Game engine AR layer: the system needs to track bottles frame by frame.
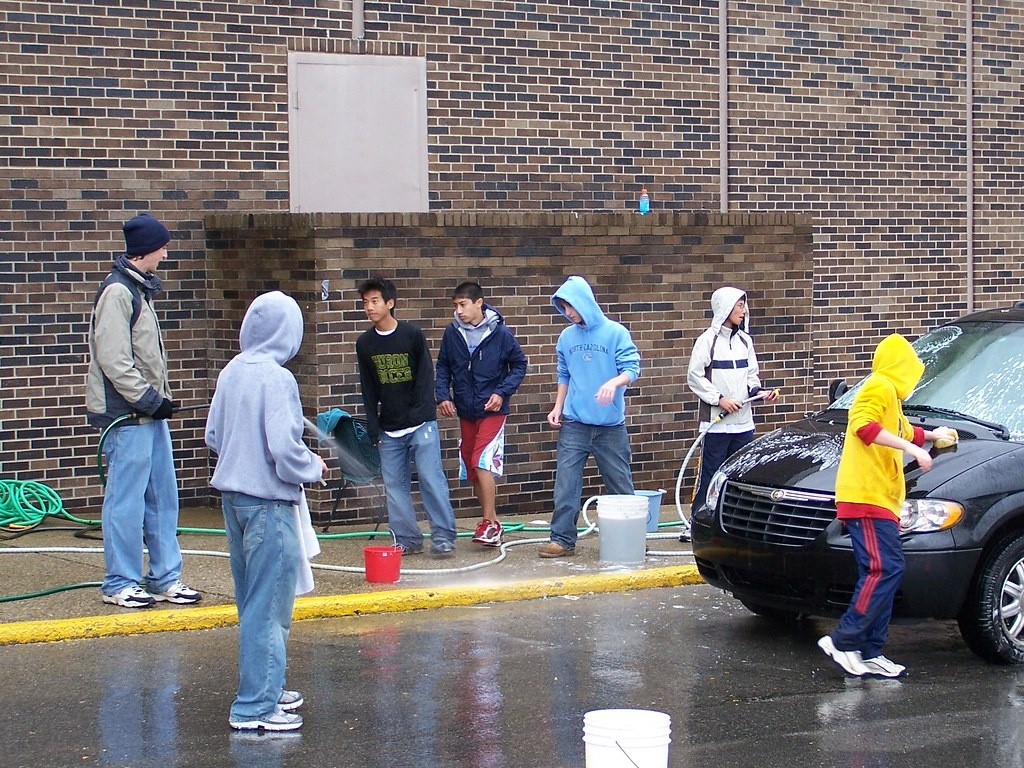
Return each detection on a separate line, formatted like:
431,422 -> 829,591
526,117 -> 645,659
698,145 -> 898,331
640,189 -> 649,213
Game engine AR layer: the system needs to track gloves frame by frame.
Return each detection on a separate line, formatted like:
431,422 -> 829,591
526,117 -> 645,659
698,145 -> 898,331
152,398 -> 180,420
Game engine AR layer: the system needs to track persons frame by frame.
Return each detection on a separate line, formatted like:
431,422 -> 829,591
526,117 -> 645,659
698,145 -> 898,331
86,213 -> 202,608
436,281 -> 527,547
679,287 -> 779,542
817,334 -> 956,677
204,290 -> 328,730
356,279 -> 456,557
538,276 -> 650,557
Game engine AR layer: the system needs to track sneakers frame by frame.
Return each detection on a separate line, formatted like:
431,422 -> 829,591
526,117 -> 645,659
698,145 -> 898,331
817,634 -> 871,676
390,539 -> 424,556
539,541 -> 576,559
275,688 -> 304,711
431,537 -> 455,554
861,653 -> 909,679
144,578 -> 204,605
101,583 -> 156,609
229,701 -> 304,730
471,518 -> 505,547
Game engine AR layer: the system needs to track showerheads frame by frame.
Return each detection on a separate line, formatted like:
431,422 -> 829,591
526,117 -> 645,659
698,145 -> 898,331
716,389 -> 781,422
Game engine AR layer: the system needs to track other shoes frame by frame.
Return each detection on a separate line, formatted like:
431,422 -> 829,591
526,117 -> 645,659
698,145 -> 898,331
679,526 -> 692,542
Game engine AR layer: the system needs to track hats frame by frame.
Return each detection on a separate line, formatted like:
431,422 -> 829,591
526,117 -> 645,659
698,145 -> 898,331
123,213 -> 171,256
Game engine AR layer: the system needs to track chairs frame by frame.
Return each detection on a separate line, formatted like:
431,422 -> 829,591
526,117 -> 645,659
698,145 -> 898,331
315,408 -> 387,541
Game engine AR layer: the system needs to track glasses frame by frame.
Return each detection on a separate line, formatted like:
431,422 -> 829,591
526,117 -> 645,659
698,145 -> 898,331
736,303 -> 746,309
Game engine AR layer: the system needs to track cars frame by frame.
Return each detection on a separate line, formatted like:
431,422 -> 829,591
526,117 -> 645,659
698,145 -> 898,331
688,300 -> 1024,667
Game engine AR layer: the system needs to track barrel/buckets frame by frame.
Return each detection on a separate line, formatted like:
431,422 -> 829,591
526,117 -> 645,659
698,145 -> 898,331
582,708 -> 672,768
596,494 -> 649,564
363,527 -> 403,584
633,487 -> 668,533
